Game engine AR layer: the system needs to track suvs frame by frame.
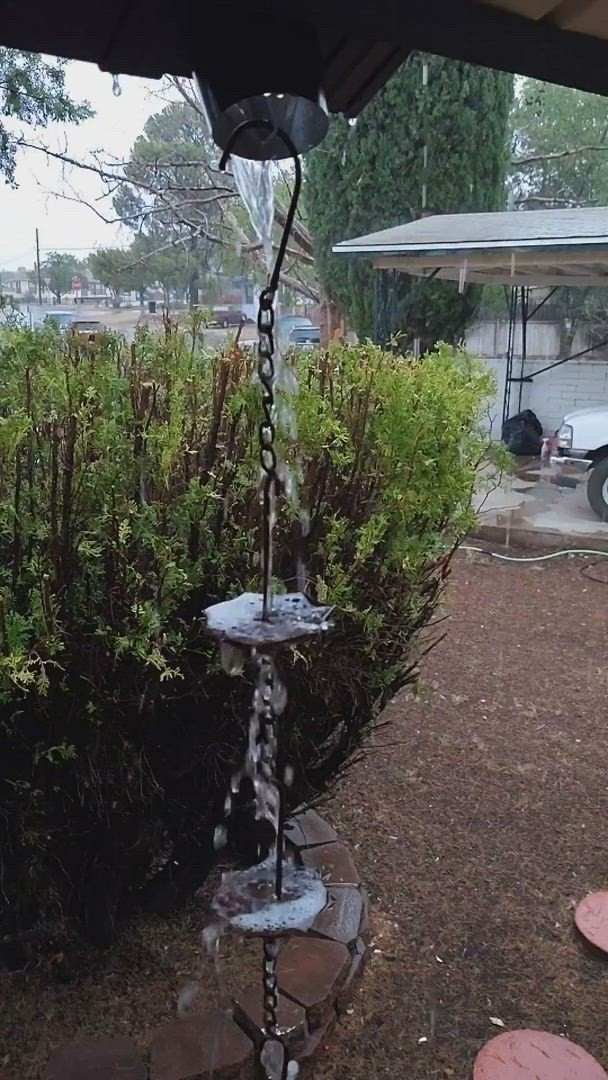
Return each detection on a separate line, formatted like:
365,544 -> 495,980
541,404 -> 608,520
288,324 -> 321,347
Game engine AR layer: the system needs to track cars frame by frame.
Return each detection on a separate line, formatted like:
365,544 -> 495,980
275,315 -> 312,340
207,304 -> 247,328
68,320 -> 104,344
34,310 -> 77,337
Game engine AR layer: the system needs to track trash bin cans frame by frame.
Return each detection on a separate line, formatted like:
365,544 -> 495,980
149,301 -> 156,313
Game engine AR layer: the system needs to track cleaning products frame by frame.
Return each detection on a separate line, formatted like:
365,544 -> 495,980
540,437 -> 549,460
